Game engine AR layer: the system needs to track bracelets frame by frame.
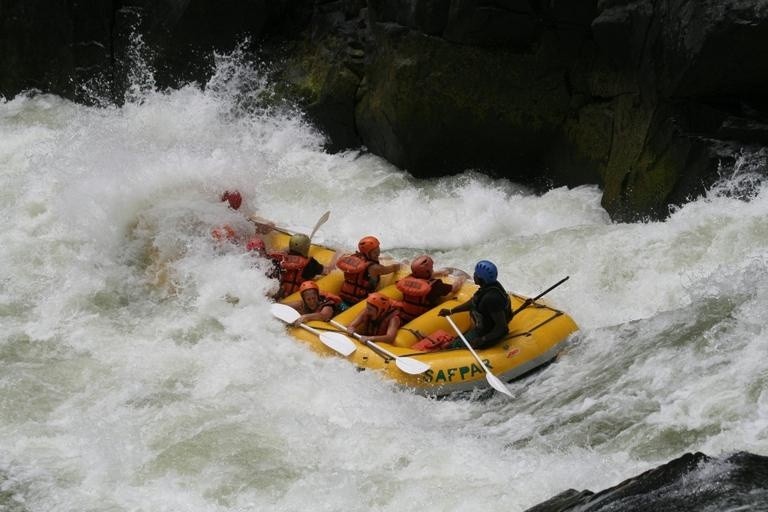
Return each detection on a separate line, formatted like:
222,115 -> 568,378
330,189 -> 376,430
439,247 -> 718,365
450,308 -> 452,314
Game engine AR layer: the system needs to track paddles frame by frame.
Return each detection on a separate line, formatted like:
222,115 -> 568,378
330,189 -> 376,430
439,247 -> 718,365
446,317 -> 517,398
300,324 -> 357,357
271,302 -> 301,323
308,211 -> 331,242
330,320 -> 431,374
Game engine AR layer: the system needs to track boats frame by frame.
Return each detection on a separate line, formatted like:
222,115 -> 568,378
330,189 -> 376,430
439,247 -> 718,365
246,216 -> 580,400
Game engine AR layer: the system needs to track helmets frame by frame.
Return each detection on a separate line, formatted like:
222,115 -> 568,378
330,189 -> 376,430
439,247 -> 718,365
411,255 -> 433,278
211,223 -> 235,242
222,187 -> 241,210
475,260 -> 498,283
287,233 -> 310,256
246,237 -> 266,250
358,236 -> 380,259
366,292 -> 389,319
299,280 -> 319,306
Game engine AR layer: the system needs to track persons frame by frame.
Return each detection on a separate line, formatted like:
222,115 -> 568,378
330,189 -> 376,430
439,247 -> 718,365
282,280 -> 344,328
210,190 -> 340,301
394,254 -> 465,321
335,235 -> 409,307
438,259 -> 516,350
346,292 -> 403,344
